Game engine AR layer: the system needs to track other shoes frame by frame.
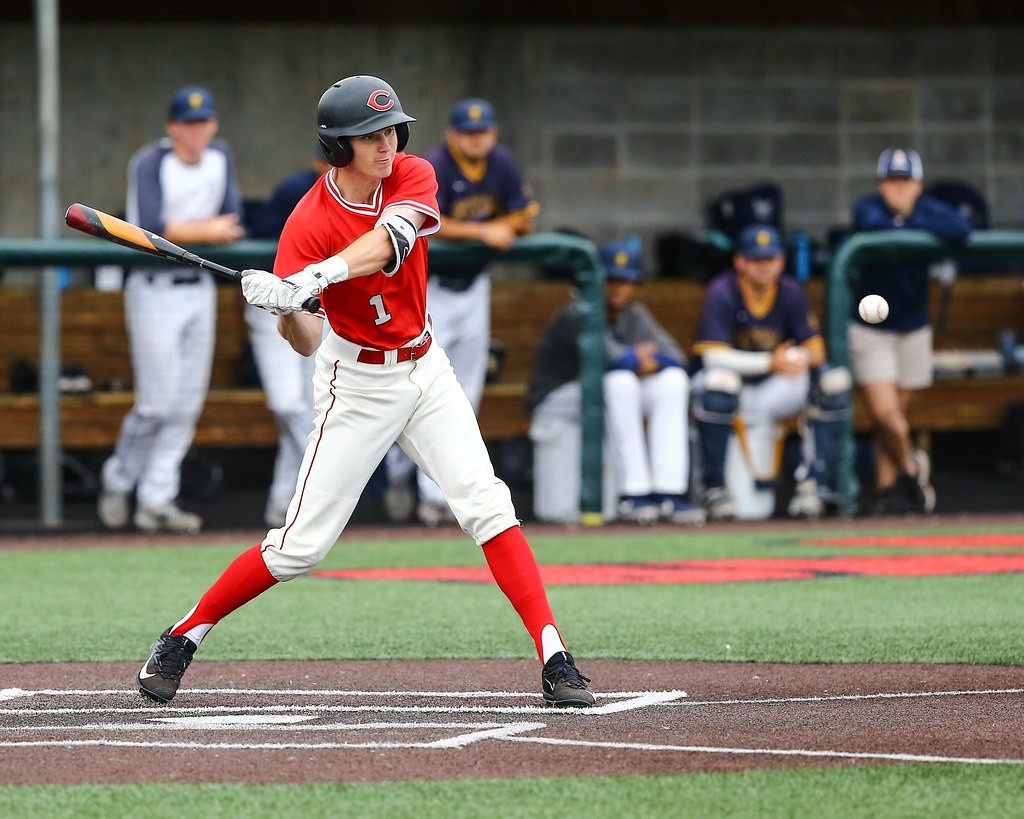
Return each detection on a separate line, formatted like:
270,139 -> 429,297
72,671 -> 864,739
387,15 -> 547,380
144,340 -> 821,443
134,505 -> 202,533
870,490 -> 909,516
905,451 -> 935,512
660,496 -> 708,523
705,488 -> 736,519
619,497 -> 660,521
98,458 -> 132,526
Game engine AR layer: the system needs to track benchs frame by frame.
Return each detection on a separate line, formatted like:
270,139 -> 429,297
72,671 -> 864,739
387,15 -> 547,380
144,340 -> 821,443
0,275 -> 1024,454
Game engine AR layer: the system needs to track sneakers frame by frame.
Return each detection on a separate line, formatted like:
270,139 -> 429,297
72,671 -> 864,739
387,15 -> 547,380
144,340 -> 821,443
542,651 -> 597,705
136,624 -> 197,704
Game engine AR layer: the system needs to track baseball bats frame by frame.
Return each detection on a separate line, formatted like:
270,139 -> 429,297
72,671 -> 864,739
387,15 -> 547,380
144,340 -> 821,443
63,200 -> 322,314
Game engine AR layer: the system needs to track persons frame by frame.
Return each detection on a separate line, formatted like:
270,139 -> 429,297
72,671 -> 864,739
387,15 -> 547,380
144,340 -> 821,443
244,145 -> 332,528
693,223 -> 825,520
97,85 -> 241,534
523,236 -> 707,525
387,98 -> 540,524
825,147 -> 974,515
137,75 -> 596,711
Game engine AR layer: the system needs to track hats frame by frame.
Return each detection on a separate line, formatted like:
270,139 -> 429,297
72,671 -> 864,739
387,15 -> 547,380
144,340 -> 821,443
738,226 -> 784,258
451,98 -> 495,131
170,87 -> 215,121
602,243 -> 647,282
877,149 -> 925,182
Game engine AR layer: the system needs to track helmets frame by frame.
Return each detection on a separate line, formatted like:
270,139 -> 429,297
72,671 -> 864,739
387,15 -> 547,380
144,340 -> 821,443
315,75 -> 417,167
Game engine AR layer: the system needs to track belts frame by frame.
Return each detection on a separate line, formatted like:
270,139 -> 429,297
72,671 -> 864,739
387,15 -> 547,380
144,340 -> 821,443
174,277 -> 199,284
440,279 -> 472,291
357,315 -> 432,365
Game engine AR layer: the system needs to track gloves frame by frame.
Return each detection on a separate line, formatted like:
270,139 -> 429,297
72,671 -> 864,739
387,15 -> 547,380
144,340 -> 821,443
240,270 -> 292,315
259,254 -> 349,312
